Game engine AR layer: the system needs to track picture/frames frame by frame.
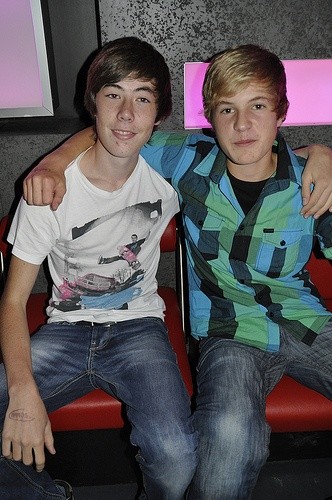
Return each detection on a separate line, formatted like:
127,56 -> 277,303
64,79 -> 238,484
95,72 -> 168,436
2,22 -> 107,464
0,0 -> 54,118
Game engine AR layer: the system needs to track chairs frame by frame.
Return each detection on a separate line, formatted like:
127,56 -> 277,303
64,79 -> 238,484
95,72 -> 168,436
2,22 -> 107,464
0,212 -> 194,435
267,237 -> 332,432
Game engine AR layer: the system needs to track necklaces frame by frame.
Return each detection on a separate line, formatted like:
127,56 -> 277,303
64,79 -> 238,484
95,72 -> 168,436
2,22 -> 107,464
22,44 -> 332,499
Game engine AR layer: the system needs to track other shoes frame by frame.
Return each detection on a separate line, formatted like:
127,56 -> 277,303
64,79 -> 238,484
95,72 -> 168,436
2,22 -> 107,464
54,478 -> 74,499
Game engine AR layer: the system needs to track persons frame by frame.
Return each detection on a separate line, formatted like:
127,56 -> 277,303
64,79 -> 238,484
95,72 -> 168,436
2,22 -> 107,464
0,37 -> 332,500
